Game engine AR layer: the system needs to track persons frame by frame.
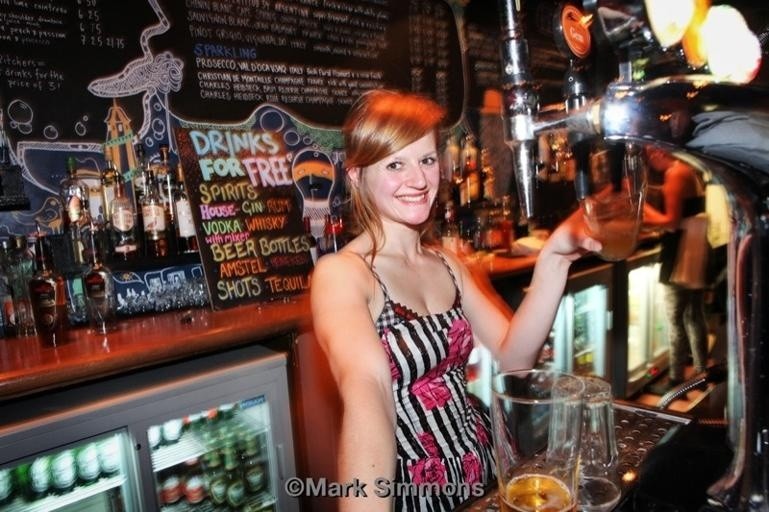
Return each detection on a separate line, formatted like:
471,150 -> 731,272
308,87 -> 613,512
624,144 -> 710,402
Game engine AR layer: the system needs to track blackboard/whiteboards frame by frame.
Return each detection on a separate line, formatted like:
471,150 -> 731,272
2,1 -> 644,214
170,128 -> 324,307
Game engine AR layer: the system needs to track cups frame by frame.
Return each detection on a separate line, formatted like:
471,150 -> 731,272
574,140 -> 648,263
487,368 -> 622,512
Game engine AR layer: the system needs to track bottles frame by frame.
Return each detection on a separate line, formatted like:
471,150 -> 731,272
0,141 -> 198,348
437,135 -> 528,251
533,131 -> 574,185
0,402 -> 276,512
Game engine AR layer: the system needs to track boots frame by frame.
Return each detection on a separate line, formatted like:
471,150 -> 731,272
647,378 -> 686,400
